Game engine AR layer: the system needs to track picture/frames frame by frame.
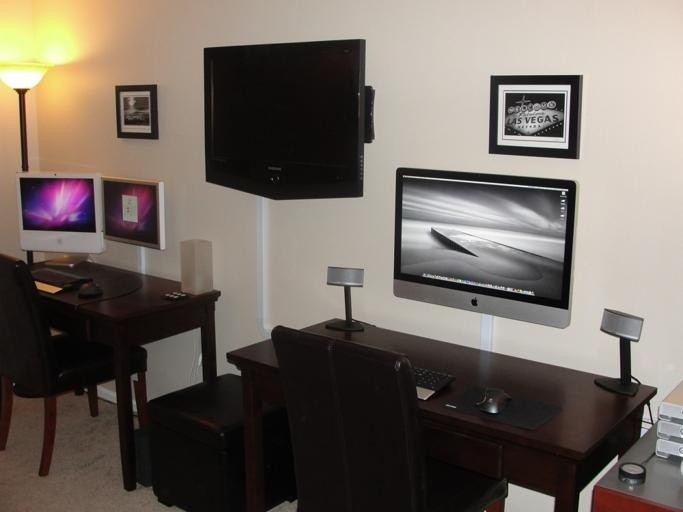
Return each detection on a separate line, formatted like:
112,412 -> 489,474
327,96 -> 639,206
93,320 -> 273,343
588,420 -> 682,511
28,256 -> 221,492
225,315 -> 659,512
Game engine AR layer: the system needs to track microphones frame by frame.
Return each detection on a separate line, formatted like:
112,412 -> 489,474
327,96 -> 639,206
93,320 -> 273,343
144,372 -> 289,512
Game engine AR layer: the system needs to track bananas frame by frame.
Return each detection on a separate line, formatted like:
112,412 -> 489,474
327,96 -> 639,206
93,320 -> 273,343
414,364 -> 456,401
31,269 -> 80,295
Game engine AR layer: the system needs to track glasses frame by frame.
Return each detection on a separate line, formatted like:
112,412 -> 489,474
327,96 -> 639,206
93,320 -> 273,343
592,307 -> 645,397
325,264 -> 367,333
0,69 -> 46,173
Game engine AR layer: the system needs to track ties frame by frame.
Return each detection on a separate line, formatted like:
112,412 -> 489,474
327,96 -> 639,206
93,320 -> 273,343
203,38 -> 374,200
394,167 -> 581,330
16,172 -> 104,268
100,176 -> 166,250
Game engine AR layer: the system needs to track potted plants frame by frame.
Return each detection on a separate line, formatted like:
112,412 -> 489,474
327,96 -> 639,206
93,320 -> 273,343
112,83 -> 160,142
486,73 -> 584,162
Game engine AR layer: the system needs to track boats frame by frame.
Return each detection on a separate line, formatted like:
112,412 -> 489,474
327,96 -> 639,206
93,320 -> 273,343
594,308 -> 644,398
325,266 -> 365,332
180,239 -> 213,295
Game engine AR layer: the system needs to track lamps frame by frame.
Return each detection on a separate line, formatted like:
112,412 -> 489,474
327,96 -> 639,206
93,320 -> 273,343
270,325 -> 511,512
0,250 -> 149,480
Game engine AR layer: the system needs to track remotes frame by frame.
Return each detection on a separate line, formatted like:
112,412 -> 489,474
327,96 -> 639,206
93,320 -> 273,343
77,282 -> 103,298
474,387 -> 512,414
